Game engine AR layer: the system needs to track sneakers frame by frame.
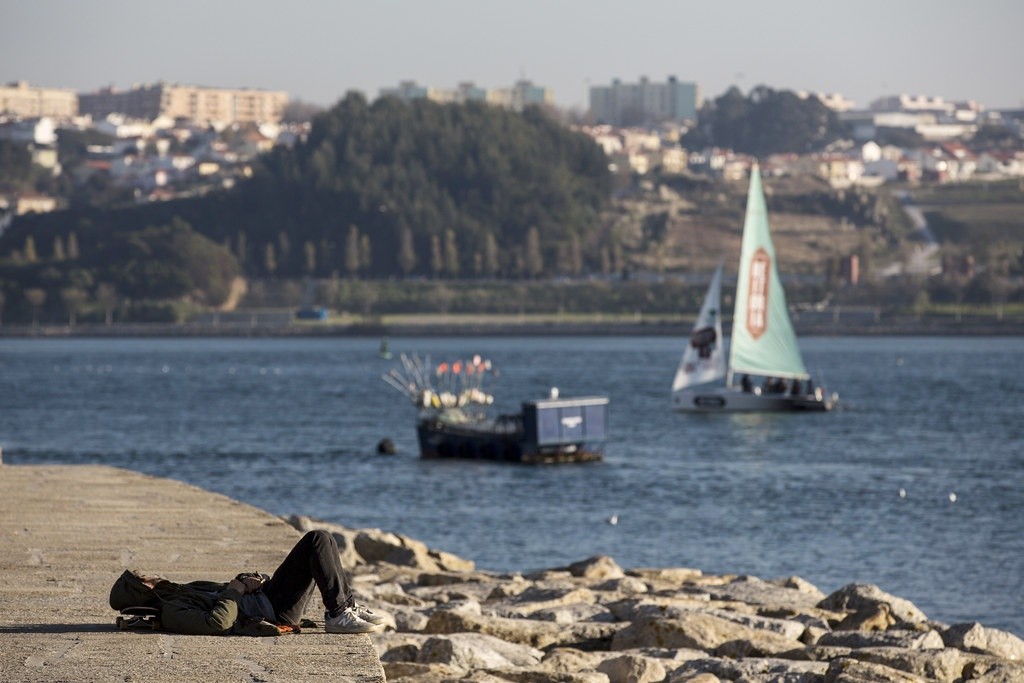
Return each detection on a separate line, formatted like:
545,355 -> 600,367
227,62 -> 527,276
350,603 -> 383,625
326,606 -> 377,633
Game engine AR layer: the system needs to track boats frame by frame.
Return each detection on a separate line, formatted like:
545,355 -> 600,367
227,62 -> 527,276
380,352 -> 608,463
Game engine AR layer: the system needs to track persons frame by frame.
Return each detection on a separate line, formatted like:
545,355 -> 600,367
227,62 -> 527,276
108,529 -> 386,638
740,374 -> 814,395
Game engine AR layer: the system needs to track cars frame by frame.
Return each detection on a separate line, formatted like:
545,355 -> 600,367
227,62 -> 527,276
295,303 -> 329,320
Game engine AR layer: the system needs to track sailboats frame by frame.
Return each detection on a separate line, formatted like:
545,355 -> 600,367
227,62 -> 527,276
669,156 -> 838,414
375,335 -> 393,360
